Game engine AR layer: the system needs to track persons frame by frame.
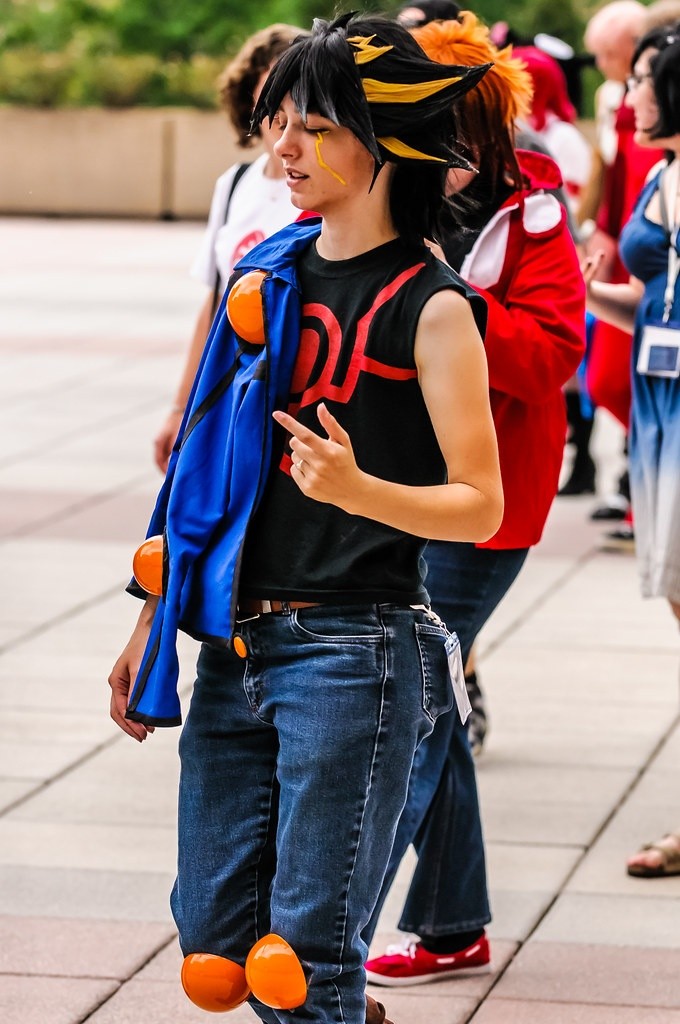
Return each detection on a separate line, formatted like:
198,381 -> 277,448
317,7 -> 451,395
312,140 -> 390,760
156,23 -> 324,474
359,11 -> 584,990
395,0 -> 680,758
584,21 -> 680,877
107,12 -> 502,1024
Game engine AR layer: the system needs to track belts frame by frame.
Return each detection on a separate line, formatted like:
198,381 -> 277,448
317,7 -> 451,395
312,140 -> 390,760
237,598 -> 320,614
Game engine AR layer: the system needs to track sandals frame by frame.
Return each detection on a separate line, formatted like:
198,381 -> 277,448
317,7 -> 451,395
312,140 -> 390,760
626,832 -> 680,874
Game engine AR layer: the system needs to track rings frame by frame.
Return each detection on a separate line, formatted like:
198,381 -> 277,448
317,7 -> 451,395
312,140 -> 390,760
296,459 -> 304,472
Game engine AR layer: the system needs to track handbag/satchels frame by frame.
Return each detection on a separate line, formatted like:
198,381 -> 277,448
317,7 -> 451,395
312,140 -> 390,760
636,324 -> 680,378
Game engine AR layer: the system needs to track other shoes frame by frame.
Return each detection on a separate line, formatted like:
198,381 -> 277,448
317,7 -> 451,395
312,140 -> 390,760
365,994 -> 395,1024
591,509 -> 633,539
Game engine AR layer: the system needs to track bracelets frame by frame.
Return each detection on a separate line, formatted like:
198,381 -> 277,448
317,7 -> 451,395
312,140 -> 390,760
167,406 -> 185,414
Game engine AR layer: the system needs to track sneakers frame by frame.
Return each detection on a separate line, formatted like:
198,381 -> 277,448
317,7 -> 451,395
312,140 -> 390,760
363,933 -> 490,986
